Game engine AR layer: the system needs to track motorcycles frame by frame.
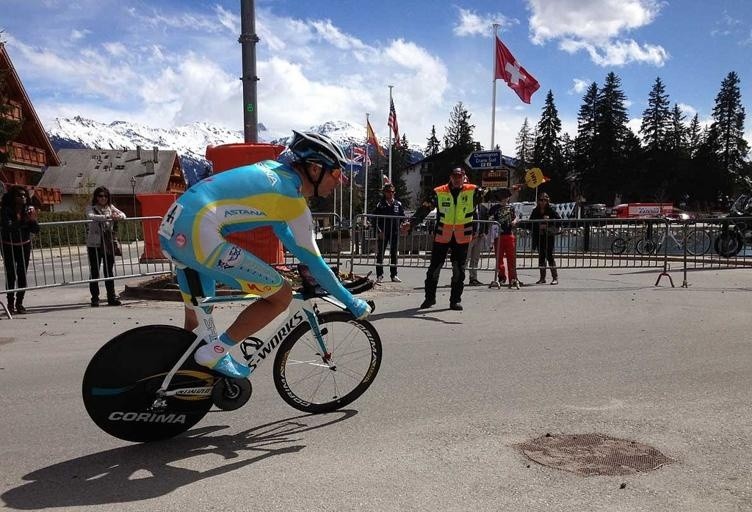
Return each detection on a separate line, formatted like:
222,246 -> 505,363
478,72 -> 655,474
711,195 -> 752,256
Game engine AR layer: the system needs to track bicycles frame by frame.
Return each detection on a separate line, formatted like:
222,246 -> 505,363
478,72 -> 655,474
79,249 -> 381,445
652,219 -> 711,256
610,226 -> 656,255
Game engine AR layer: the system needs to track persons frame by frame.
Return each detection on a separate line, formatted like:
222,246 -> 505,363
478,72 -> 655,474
466,187 -> 490,285
85,186 -> 127,307
526,192 -> 563,284
370,183 -> 406,283
401,167 -> 485,311
488,188 -> 524,286
729,193 -> 752,232
0,185 -> 40,315
159,129 -> 373,379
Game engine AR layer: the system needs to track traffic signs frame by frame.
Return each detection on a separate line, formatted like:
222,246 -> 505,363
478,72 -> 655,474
463,149 -> 503,170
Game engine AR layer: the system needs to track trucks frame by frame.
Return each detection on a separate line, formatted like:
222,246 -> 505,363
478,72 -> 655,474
398,198 -> 697,226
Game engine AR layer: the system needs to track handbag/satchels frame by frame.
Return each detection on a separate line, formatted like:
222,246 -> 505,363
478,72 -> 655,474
102,230 -> 122,256
539,223 -> 562,235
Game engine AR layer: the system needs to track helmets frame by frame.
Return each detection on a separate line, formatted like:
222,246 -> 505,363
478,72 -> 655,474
289,129 -> 347,170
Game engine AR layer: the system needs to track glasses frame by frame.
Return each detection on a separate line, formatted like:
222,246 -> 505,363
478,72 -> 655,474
306,158 -> 342,179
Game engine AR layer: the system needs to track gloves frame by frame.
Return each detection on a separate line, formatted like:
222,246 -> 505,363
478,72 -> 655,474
348,298 -> 372,321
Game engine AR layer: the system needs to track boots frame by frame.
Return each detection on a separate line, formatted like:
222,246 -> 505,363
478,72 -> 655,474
193,332 -> 245,379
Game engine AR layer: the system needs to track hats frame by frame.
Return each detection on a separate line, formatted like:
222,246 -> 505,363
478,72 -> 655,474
450,167 -> 466,175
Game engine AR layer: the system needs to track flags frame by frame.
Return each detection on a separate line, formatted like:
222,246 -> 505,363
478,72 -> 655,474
495,35 -> 541,104
337,97 -> 400,187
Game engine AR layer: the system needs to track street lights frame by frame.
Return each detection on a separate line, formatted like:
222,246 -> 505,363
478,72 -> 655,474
128,176 -> 140,242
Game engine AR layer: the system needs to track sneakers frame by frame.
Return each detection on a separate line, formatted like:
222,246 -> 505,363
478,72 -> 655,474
470,279 -> 483,286
8,304 -> 14,315
91,301 -> 99,306
551,279 -> 558,284
536,278 -> 546,284
376,276 -> 383,282
392,276 -> 402,283
107,299 -> 121,306
421,299 -> 436,309
15,302 -> 27,314
450,302 -> 463,310
